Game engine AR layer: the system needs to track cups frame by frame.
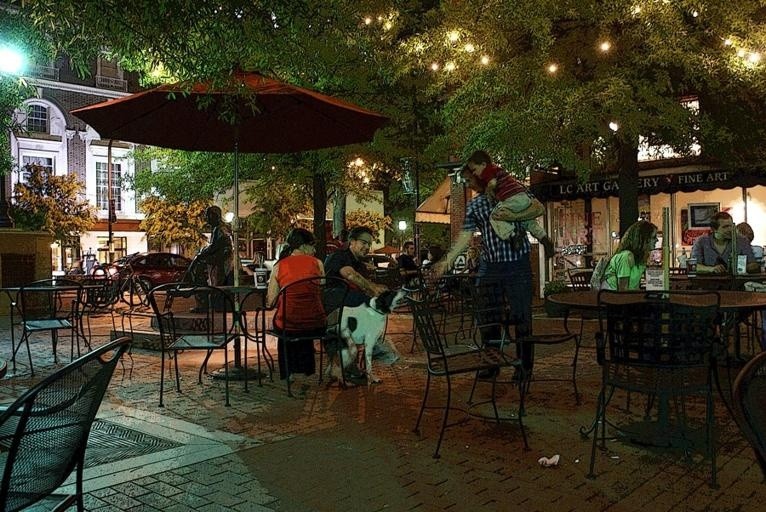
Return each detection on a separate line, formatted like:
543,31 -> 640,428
253,267 -> 268,290
686,258 -> 697,278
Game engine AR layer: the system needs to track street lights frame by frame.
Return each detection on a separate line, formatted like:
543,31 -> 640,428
399,220 -> 406,247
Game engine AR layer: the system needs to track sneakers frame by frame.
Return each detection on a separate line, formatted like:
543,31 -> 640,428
478,365 -> 523,382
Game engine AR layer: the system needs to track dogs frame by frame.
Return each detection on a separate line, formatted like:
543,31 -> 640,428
325,288 -> 411,384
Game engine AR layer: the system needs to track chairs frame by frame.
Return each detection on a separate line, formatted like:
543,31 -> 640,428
146,280 -> 241,409
78,257 -> 764,359
1,336 -> 132,511
732,351 -> 762,478
8,278 -> 90,378
468,283 -> 582,418
587,288 -> 721,493
484,258 -> 582,406
253,274 -> 350,397
401,281 -> 532,461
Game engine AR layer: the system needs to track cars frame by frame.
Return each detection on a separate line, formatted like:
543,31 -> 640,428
241,264 -> 272,283
90,253 -> 192,294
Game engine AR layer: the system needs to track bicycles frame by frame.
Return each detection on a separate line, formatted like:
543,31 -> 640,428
77,252 -> 153,308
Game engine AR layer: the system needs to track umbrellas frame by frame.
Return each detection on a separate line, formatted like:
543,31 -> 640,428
70,63 -> 391,364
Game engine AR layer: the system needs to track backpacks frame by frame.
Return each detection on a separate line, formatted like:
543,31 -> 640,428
589,249 -> 636,289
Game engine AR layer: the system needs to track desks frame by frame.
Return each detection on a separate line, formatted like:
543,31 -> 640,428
546,284 -> 764,456
167,287 -> 269,382
1,285 -> 106,373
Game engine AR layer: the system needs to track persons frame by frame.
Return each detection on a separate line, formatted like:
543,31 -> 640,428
690,210 -> 760,350
600,220 -> 659,292
267,227 -> 328,388
171,205 -> 243,314
736,222 -> 766,276
410,245 -> 443,308
320,225 -> 391,383
398,242 -> 426,299
430,164 -> 547,382
466,150 -> 554,257
465,244 -> 479,298
